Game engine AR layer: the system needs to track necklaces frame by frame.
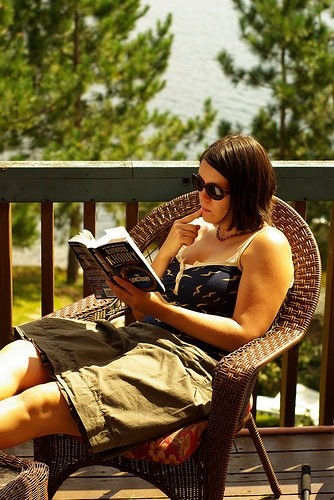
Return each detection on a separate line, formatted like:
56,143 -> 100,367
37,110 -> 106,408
216,225 -> 249,241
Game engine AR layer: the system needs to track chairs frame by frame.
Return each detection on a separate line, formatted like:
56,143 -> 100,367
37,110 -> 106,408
0,452 -> 49,500
28,188 -> 321,500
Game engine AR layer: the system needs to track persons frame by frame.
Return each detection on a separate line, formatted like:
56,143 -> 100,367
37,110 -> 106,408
0,135 -> 294,455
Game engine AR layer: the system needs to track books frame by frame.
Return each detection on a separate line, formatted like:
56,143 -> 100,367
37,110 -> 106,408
68,225 -> 166,300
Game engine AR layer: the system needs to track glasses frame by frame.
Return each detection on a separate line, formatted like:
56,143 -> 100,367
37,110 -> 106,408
192,172 -> 231,200
